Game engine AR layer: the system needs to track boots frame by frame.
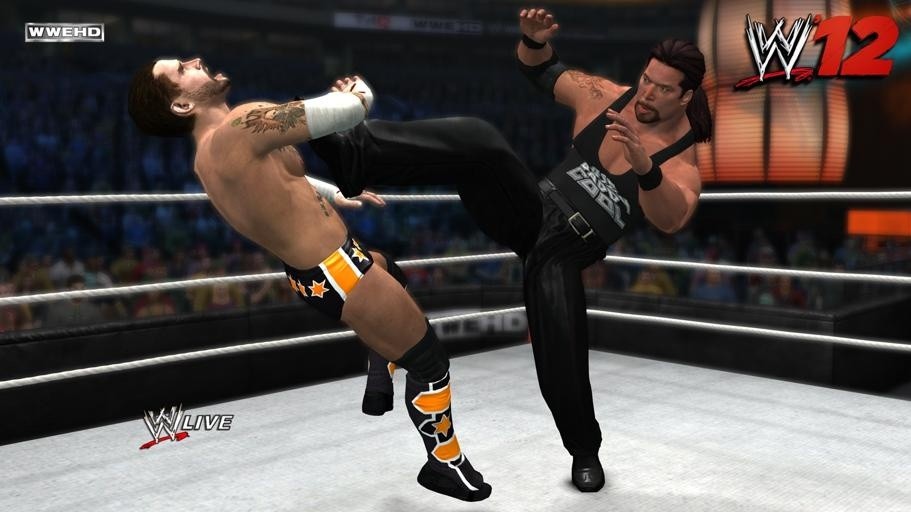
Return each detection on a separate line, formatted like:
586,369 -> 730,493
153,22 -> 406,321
402,370 -> 493,504
360,346 -> 397,416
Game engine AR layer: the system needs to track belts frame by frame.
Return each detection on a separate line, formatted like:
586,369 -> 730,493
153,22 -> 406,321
536,177 -> 606,262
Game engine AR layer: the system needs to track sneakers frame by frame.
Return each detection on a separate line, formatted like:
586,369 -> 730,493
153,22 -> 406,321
568,452 -> 606,495
289,93 -> 366,201
